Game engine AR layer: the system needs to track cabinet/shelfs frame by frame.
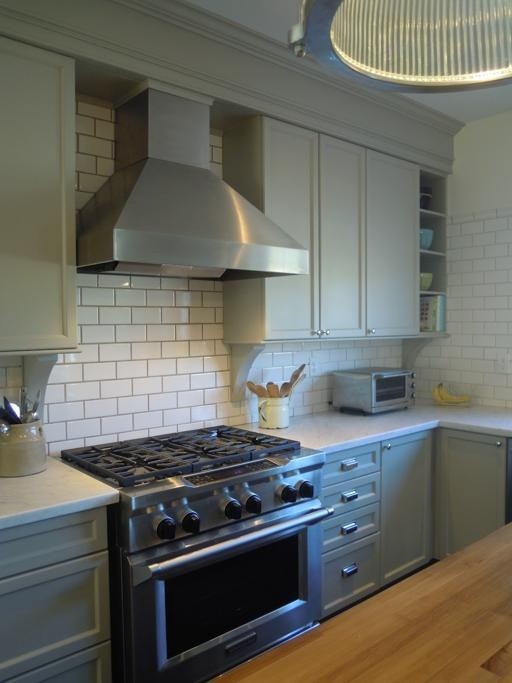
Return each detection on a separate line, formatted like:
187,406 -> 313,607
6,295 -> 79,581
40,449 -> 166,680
325,443 -> 385,624
363,146 -> 420,337
435,428 -> 508,561
222,115 -> 363,346
0,36 -> 81,353
381,430 -> 436,586
418,166 -> 451,338
1,515 -> 120,683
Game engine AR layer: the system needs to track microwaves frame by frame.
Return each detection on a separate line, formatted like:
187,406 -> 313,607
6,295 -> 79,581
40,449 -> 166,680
333,365 -> 417,413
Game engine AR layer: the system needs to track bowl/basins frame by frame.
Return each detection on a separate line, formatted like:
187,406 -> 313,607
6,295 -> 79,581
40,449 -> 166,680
420,272 -> 433,290
419,227 -> 434,249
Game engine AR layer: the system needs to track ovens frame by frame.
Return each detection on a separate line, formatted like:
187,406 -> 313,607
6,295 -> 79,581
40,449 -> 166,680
111,450 -> 334,683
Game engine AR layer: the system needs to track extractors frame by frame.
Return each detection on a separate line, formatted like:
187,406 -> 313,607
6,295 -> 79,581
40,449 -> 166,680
77,88 -> 311,271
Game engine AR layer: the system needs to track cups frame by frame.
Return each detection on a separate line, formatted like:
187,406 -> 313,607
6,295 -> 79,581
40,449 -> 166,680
258,397 -> 290,428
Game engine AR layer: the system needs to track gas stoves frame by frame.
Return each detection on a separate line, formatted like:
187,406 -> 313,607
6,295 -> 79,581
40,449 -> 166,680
60,423 -> 299,484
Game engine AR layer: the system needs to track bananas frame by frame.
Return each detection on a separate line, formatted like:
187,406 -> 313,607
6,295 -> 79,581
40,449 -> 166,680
431,383 -> 469,406
